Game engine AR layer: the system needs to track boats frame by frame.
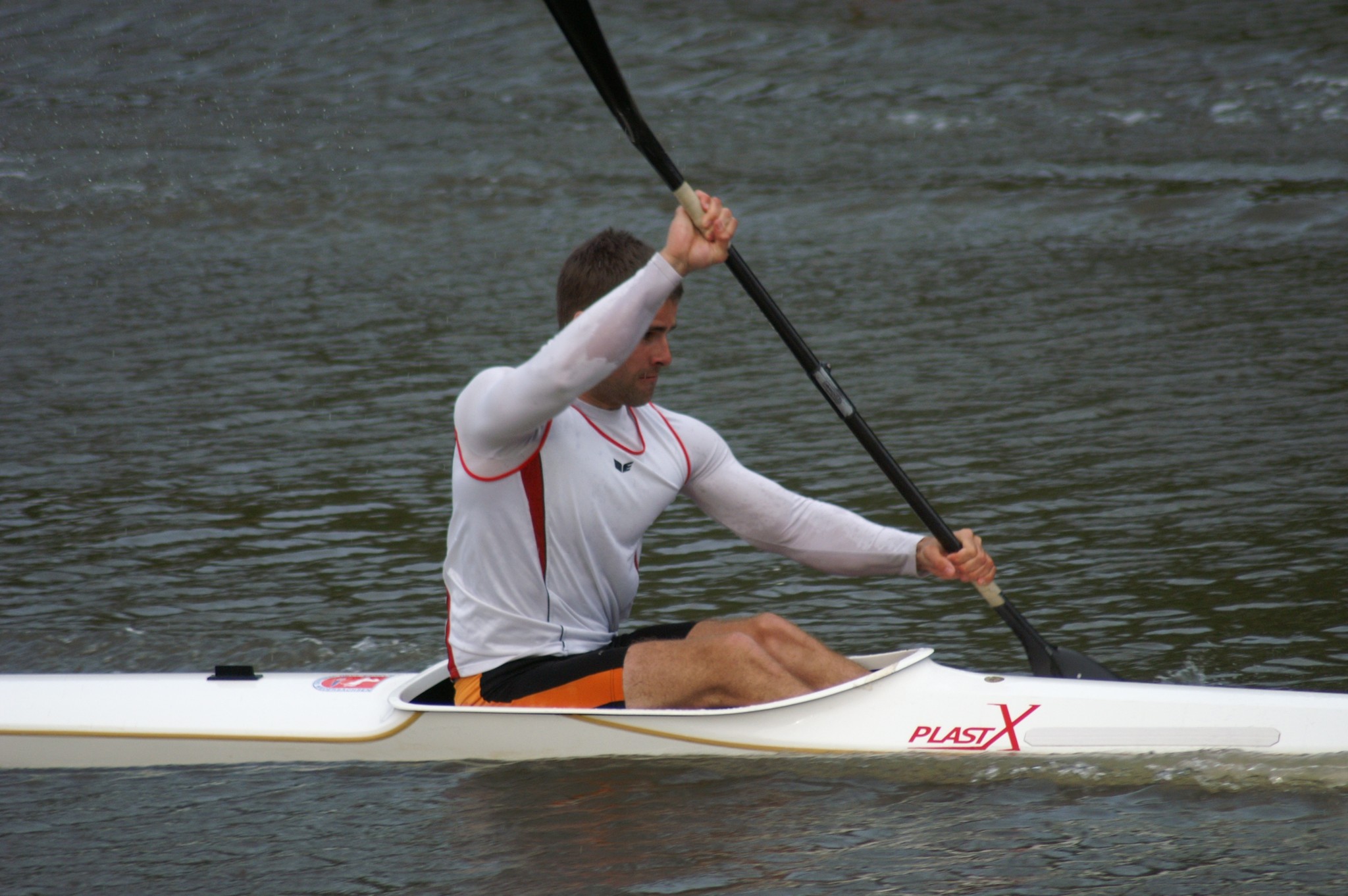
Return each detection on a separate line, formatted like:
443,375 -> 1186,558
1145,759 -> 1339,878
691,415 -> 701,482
1,646 -> 1348,795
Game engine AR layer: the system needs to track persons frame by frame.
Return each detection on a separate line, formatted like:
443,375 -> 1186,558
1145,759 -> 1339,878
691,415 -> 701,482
443,189 -> 997,709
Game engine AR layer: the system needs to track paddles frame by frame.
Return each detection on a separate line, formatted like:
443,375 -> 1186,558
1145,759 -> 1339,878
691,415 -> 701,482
544,1 -> 1122,681
